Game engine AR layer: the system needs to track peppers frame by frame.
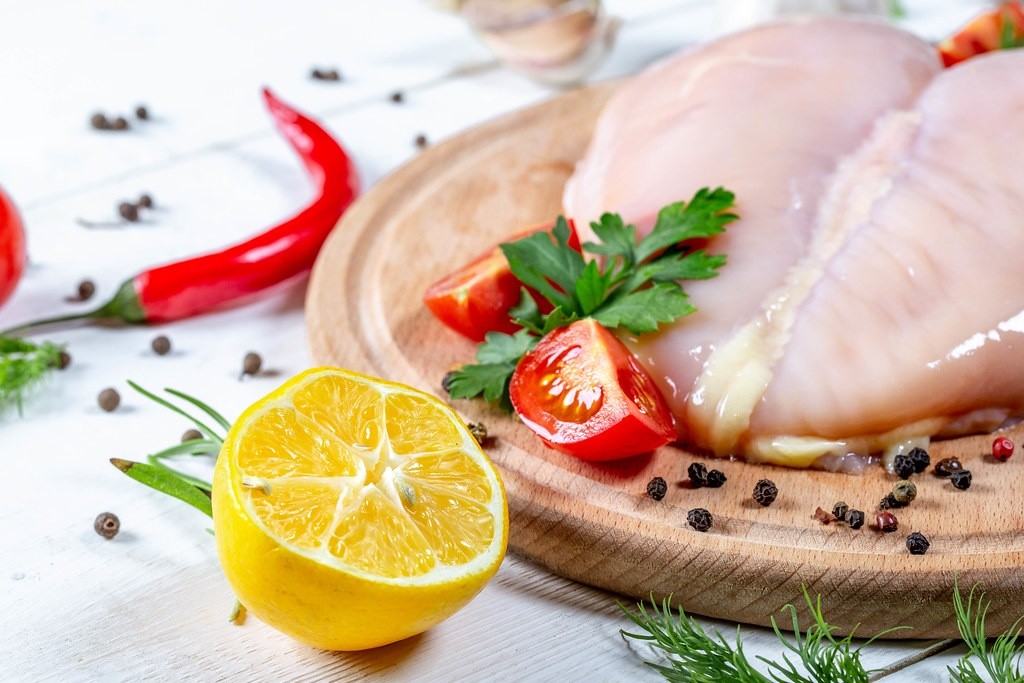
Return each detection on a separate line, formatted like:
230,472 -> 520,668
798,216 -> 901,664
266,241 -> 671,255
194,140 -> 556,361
0,84 -> 362,339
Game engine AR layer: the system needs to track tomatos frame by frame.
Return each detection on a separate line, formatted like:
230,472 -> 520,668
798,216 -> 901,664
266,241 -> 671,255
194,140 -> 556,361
425,216 -> 676,462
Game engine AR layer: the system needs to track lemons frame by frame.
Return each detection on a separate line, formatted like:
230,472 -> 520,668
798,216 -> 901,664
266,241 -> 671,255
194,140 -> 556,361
213,365 -> 512,654
